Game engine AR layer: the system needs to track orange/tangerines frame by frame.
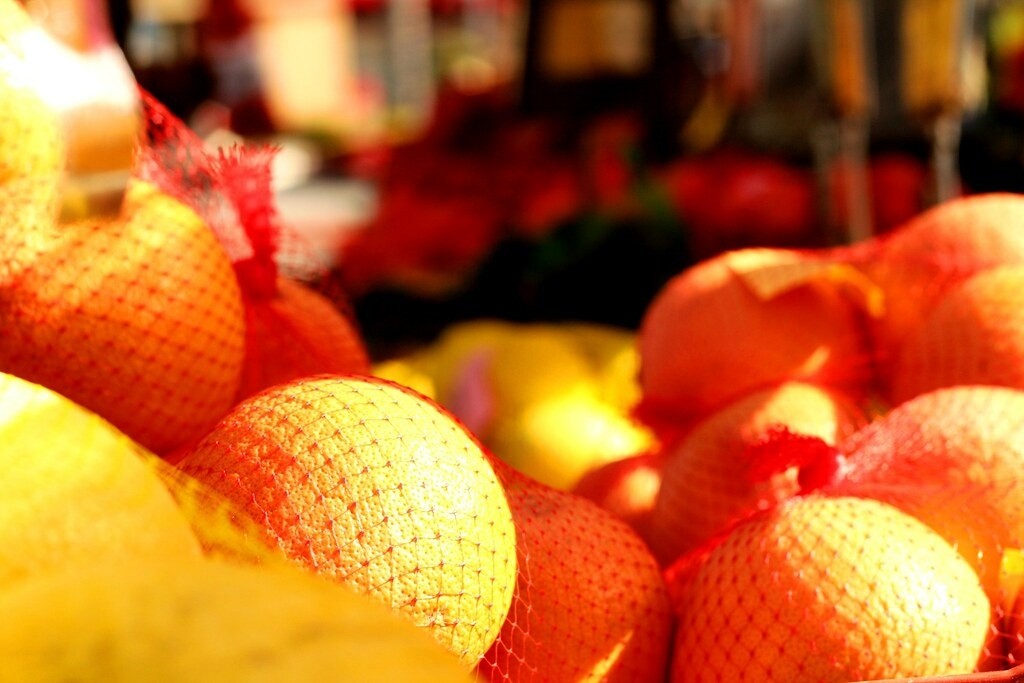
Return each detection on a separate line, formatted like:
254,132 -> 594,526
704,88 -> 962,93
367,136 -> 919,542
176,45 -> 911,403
0,1 -> 1024,682
